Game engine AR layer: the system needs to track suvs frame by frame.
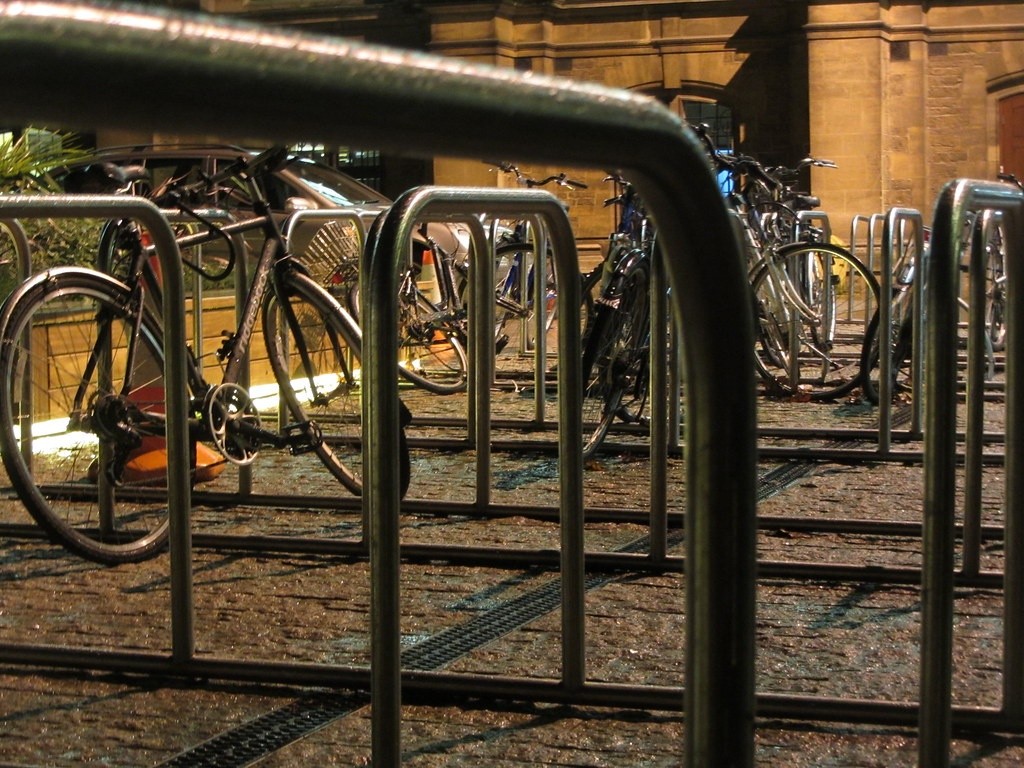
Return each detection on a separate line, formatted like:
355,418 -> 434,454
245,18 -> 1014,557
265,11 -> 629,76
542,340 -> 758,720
34,141 -> 529,282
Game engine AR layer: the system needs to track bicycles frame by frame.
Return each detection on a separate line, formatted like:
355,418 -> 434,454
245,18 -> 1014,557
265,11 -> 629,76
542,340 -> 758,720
0,121 -> 1023,567
